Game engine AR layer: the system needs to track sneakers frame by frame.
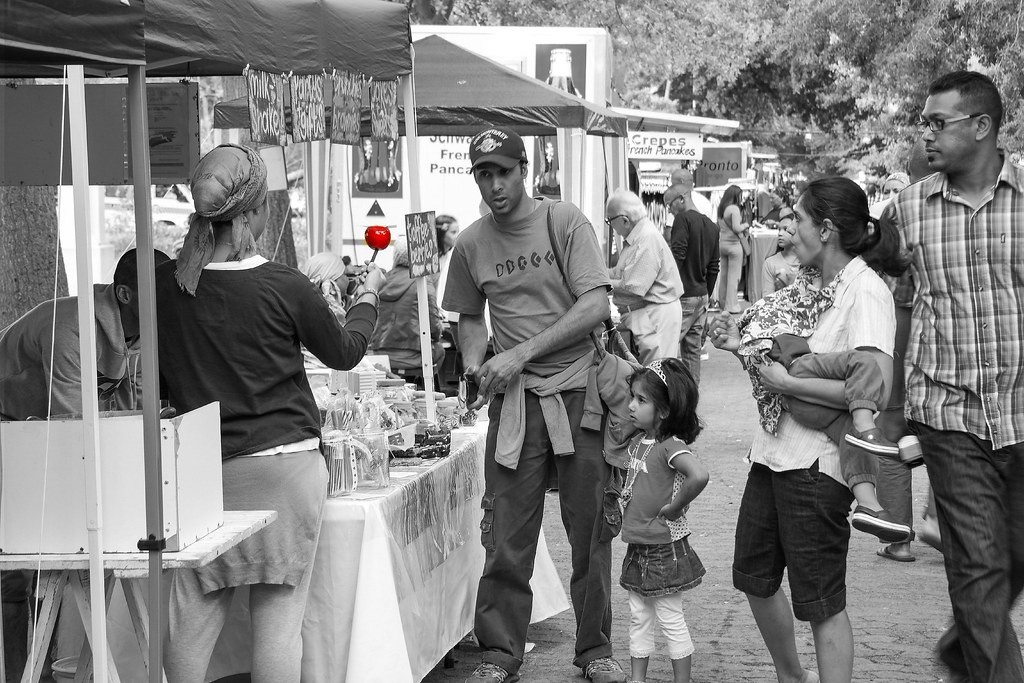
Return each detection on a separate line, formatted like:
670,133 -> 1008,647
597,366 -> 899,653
465,663 -> 520,683
583,656 -> 627,683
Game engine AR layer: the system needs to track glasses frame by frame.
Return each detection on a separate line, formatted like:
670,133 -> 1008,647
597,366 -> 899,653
917,112 -> 983,133
604,215 -> 627,224
665,196 -> 681,211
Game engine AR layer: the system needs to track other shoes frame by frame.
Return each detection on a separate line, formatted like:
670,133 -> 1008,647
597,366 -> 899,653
707,300 -> 720,312
730,310 -> 743,314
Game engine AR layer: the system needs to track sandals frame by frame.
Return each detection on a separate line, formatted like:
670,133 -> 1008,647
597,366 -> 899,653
852,506 -> 911,542
845,426 -> 900,456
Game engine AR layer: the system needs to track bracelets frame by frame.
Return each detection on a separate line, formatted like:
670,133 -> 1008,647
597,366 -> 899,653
355,289 -> 380,304
618,434 -> 659,516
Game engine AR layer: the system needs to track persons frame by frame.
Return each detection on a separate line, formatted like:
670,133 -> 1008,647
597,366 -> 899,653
152,144 -> 387,683
707,177 -> 911,683
618,358 -> 710,683
877,72 -> 1024,683
607,189 -> 683,364
443,130 -> 628,683
299,216 -> 459,368
663,170 -> 721,391
0,249 -> 171,683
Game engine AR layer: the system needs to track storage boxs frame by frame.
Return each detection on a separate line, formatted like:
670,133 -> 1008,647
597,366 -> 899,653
0,399 -> 225,554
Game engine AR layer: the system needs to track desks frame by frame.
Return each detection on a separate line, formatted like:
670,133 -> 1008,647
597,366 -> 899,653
117,418 -> 571,683
0,508 -> 278,682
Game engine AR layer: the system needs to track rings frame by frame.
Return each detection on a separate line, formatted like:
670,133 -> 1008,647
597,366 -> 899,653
713,330 -> 718,336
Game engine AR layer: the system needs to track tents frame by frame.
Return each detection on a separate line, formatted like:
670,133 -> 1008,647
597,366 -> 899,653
0,0 -> 628,681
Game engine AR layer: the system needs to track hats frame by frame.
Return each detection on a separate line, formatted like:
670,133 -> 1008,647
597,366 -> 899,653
470,126 -> 528,174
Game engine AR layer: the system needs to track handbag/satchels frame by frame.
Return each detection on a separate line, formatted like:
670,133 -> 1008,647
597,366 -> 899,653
739,234 -> 752,256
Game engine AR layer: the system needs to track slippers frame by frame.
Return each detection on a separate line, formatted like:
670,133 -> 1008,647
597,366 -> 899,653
919,517 -> 942,553
877,546 -> 915,563
